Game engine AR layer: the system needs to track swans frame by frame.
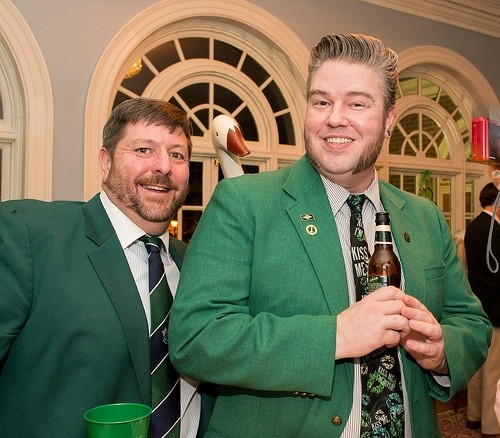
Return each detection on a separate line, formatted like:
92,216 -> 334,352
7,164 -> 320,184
210,113 -> 252,180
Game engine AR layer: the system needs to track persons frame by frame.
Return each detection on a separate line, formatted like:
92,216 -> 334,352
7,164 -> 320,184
170,34 -> 493,438
465,183 -> 500,438
0,98 -> 219,438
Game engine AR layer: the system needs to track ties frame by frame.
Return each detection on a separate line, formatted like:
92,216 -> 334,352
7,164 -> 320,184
346,193 -> 406,438
138,235 -> 181,438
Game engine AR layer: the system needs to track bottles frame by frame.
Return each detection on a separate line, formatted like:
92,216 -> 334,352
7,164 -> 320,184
367,211 -> 402,295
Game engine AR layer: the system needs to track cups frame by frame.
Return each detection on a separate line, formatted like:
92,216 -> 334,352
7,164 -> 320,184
84,402 -> 152,438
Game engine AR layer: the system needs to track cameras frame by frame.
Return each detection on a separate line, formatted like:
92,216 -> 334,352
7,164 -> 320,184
471,116 -> 500,165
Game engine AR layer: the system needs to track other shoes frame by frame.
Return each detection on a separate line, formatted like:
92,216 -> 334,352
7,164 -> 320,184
482,433 -> 500,438
466,419 -> 481,430
